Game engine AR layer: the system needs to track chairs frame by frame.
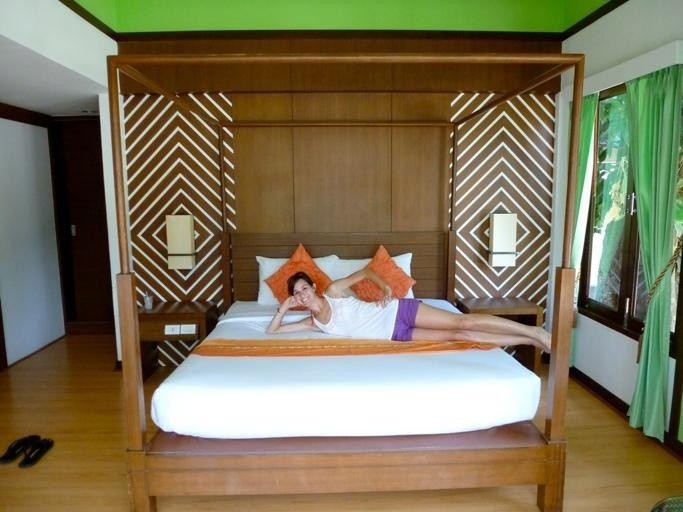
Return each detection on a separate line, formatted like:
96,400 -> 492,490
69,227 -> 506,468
649,495 -> 683,512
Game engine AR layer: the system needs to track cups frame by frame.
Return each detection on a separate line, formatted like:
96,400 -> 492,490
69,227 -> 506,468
144,295 -> 153,310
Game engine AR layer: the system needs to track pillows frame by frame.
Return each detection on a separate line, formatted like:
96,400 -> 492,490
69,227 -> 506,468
255,242 -> 417,310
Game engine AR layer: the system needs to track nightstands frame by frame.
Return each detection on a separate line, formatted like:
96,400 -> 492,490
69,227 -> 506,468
455,296 -> 543,377
138,301 -> 217,382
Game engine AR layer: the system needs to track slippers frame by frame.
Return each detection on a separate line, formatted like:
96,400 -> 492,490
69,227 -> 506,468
0,433 -> 41,464
18,438 -> 54,467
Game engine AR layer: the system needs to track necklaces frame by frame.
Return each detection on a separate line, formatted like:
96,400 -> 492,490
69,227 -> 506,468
325,320 -> 328,323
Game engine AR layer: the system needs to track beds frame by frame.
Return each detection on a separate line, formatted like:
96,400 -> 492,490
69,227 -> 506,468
104,52 -> 586,512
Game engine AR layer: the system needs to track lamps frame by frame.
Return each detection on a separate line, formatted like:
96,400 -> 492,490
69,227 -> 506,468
164,214 -> 196,270
488,212 -> 518,267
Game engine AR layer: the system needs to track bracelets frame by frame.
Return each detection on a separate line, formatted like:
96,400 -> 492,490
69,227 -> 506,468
276,308 -> 281,314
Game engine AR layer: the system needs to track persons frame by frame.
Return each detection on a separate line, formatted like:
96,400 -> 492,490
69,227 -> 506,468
264,267 -> 552,354
